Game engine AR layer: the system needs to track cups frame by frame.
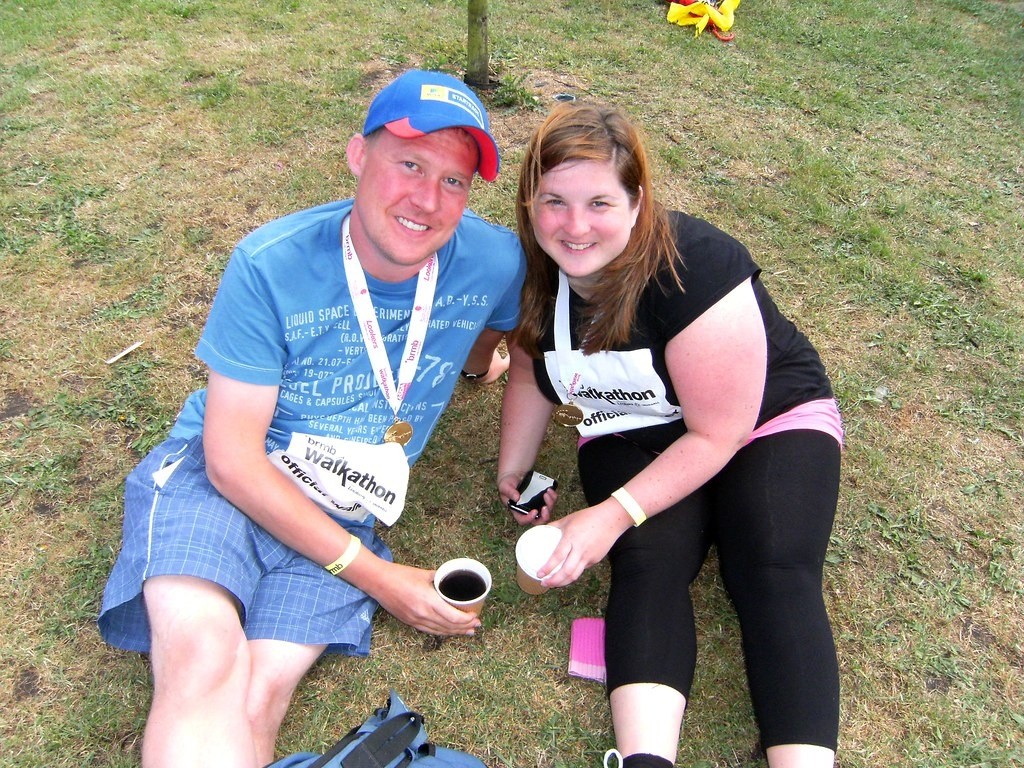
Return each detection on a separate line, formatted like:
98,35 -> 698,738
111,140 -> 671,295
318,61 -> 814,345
515,526 -> 567,596
433,558 -> 492,618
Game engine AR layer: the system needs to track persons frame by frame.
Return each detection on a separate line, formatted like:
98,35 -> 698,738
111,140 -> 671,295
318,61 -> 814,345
97,70 -> 525,768
496,98 -> 843,766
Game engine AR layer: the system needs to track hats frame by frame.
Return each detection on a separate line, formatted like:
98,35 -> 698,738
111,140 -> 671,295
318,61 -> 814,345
363,70 -> 499,182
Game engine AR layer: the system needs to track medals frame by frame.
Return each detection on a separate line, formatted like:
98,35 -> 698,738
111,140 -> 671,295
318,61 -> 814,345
554,404 -> 583,427
384,421 -> 413,447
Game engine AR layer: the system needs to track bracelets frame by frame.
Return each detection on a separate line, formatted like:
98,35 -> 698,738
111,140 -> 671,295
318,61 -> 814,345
326,535 -> 361,575
460,369 -> 488,380
611,487 -> 646,527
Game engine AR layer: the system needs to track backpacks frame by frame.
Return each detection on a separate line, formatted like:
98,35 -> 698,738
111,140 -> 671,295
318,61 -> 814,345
261,688 -> 489,768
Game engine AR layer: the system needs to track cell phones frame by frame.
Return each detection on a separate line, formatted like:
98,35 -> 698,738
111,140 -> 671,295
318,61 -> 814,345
507,469 -> 559,518
